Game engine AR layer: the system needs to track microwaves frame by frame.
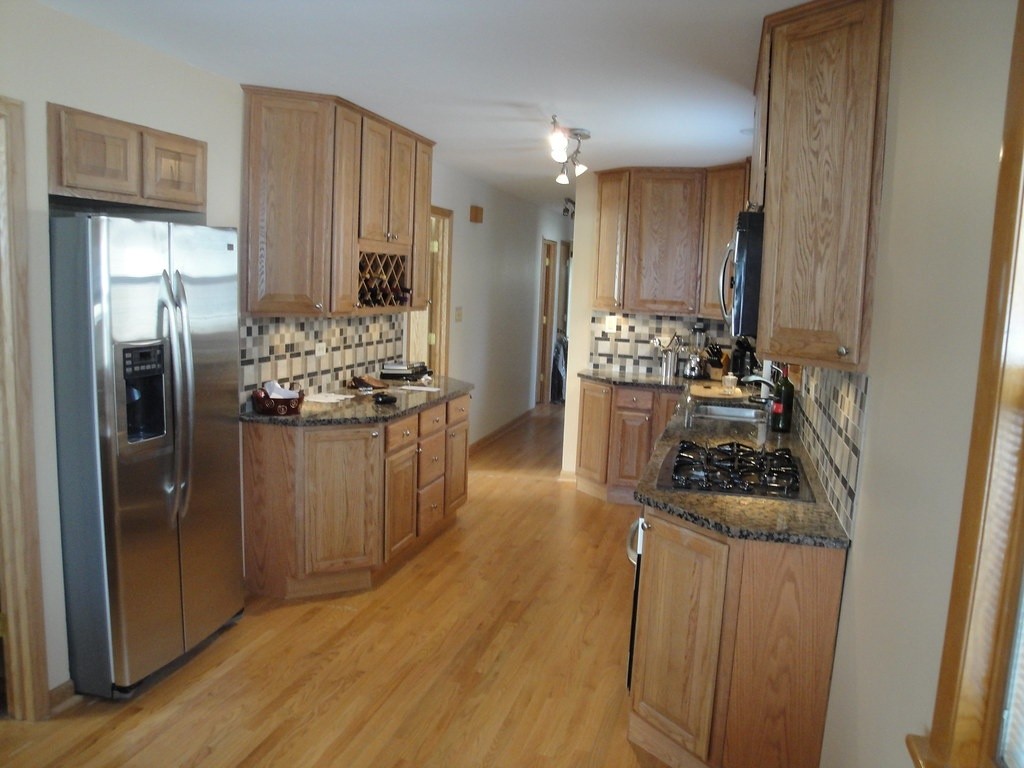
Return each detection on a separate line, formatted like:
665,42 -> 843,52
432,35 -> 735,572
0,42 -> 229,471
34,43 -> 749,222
718,212 -> 764,339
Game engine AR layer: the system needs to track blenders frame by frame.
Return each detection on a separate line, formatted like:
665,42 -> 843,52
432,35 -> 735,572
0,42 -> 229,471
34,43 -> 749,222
683,327 -> 706,379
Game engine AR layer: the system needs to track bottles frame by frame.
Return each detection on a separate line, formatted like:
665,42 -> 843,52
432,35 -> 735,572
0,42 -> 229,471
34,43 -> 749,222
770,366 -> 794,434
721,372 -> 738,395
358,272 -> 412,306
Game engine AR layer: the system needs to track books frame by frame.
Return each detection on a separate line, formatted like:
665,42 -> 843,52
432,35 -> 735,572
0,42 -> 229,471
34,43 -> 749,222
380,362 -> 433,381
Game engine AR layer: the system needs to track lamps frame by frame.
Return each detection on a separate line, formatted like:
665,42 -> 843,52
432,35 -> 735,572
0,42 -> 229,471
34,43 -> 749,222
550,116 -> 590,185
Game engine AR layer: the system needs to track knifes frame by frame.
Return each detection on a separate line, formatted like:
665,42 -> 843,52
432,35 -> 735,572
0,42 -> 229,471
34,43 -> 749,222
705,342 -> 723,359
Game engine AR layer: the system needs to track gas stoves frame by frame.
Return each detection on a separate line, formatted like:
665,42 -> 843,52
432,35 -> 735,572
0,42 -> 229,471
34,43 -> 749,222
656,439 -> 817,503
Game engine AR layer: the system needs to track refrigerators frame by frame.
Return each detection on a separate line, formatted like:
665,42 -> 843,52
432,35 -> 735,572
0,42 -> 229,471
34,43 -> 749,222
49,211 -> 244,701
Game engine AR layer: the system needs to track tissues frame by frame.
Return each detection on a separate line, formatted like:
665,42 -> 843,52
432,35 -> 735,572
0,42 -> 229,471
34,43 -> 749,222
251,381 -> 305,416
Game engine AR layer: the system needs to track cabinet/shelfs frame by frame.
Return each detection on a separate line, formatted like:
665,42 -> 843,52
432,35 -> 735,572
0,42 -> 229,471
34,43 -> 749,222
593,0 -> 894,371
575,378 -> 686,506
239,84 -> 437,318
242,394 -> 471,611
45,103 -> 208,213
626,503 -> 847,768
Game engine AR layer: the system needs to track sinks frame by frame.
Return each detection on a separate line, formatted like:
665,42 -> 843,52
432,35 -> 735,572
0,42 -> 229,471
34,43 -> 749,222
692,404 -> 767,423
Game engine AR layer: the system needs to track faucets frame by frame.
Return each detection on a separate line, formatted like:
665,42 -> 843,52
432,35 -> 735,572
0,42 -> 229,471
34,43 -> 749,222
741,372 -> 776,392
